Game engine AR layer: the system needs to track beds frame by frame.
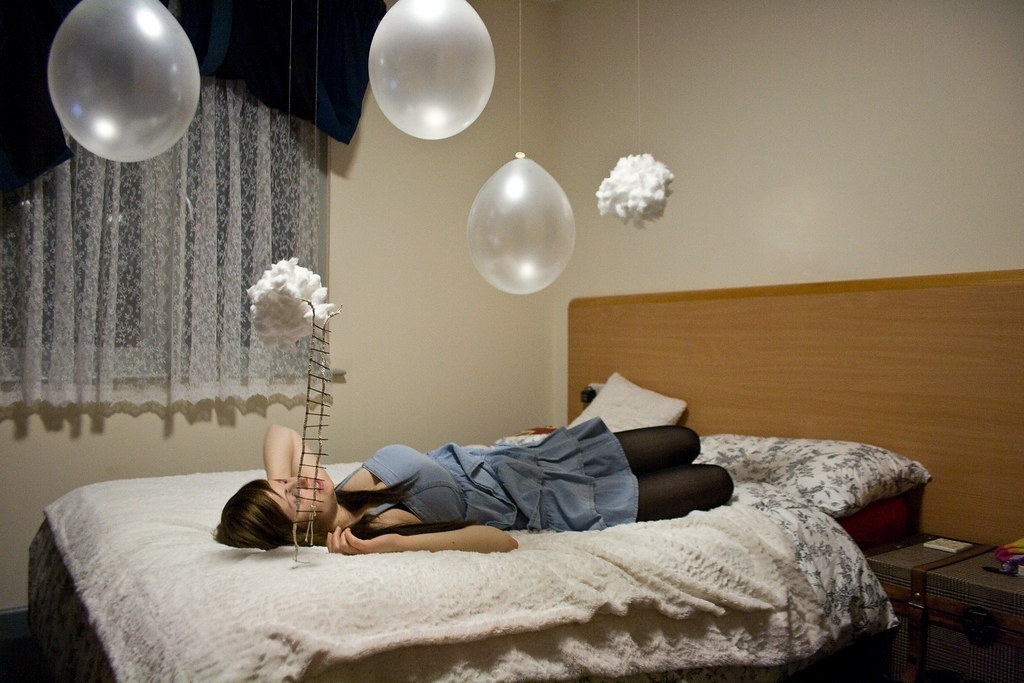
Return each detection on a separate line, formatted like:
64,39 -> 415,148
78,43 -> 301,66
27,259 -> 1024,683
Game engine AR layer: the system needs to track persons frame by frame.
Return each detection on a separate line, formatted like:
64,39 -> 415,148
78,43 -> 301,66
216,415 -> 735,556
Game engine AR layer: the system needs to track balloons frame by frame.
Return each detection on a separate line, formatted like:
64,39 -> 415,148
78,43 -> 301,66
368,0 -> 496,139
464,152 -> 577,294
48,1 -> 202,164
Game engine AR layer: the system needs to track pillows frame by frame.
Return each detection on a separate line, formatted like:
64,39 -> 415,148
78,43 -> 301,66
565,373 -> 688,433
694,434 -> 931,519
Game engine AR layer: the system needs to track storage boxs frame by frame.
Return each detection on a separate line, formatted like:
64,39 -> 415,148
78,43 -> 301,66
863,535 -> 1024,683
